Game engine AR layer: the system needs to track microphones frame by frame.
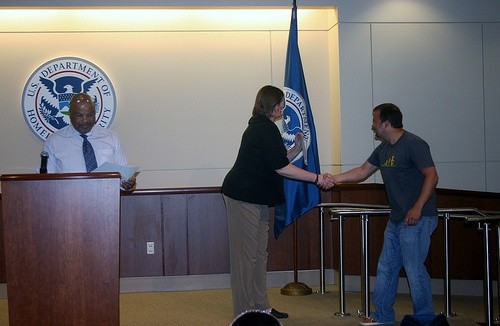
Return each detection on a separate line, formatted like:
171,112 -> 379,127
40,151 -> 49,174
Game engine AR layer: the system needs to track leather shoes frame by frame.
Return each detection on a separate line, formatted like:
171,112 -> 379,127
270,309 -> 288,318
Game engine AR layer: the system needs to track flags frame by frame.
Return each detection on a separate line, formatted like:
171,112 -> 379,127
273,10 -> 322,240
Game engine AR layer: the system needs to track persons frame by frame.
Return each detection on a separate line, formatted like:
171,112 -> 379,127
219,85 -> 335,326
320,103 -> 439,326
44,93 -> 141,193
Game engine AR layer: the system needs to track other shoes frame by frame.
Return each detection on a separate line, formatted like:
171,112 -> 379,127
360,316 -> 385,324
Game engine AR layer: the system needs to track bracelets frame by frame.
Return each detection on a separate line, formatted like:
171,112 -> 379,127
311,174 -> 320,185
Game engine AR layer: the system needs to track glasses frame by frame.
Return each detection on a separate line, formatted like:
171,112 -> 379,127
71,98 -> 93,104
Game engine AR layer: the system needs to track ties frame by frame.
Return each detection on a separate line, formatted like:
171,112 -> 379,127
80,134 -> 98,172
